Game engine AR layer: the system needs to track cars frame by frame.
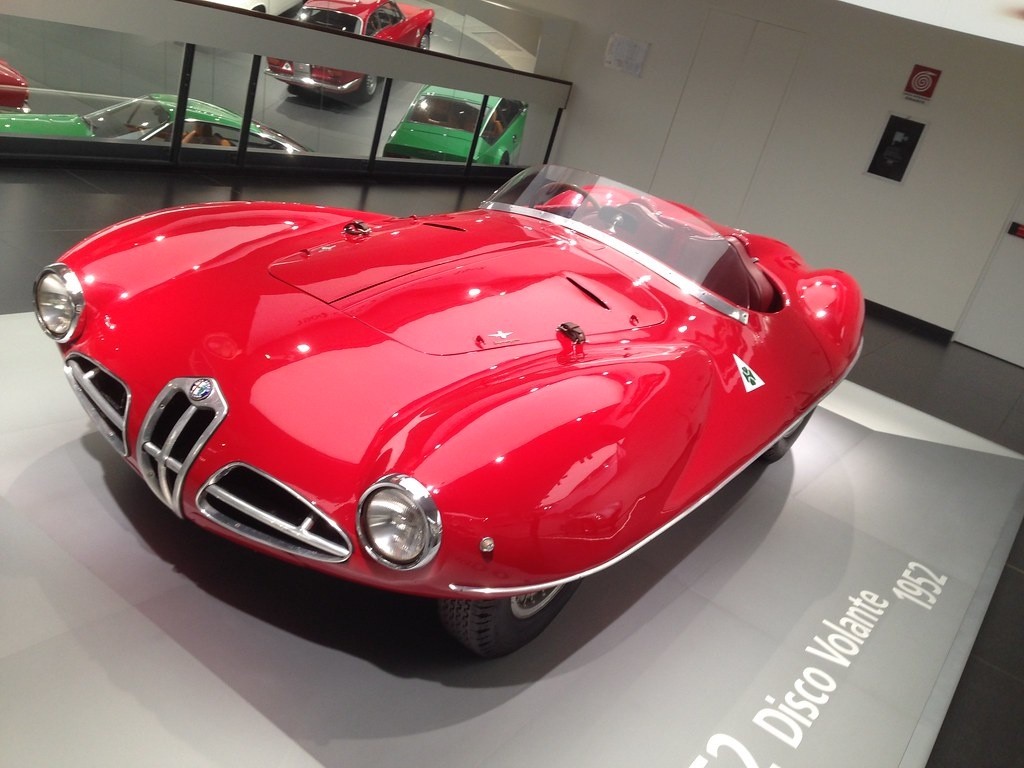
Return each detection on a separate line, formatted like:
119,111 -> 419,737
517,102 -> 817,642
0,89 -> 317,156
377,79 -> 531,166
39,160 -> 865,660
0,58 -> 29,109
265,0 -> 436,103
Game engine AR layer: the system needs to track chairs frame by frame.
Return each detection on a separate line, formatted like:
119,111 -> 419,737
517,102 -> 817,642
464,109 -> 503,145
579,202 -> 674,264
682,236 -> 773,314
182,121 -> 231,146
